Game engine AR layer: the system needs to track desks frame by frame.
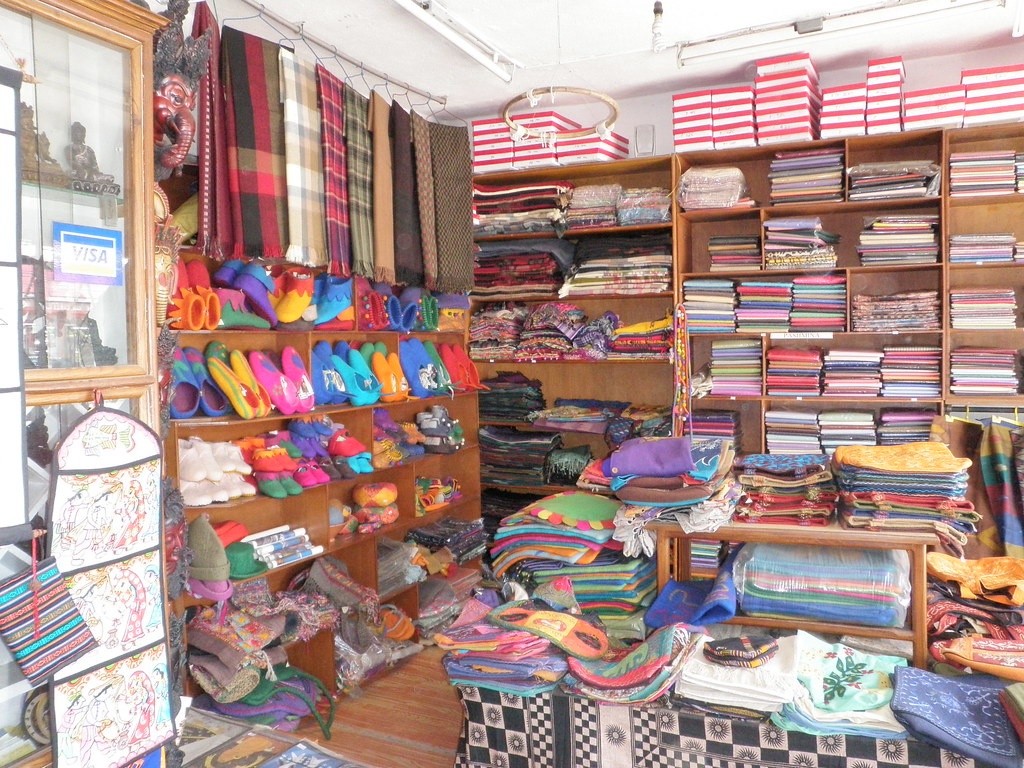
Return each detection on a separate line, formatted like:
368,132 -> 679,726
446,681 -> 992,768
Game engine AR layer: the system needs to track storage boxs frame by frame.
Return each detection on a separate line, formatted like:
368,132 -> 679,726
470,111 -> 630,177
670,51 -> 1024,152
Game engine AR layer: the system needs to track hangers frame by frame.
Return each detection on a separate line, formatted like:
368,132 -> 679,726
279,24 -> 325,69
393,82 -> 412,108
343,61 -> 371,100
425,97 -> 469,128
411,92 -> 440,125
223,3 -> 295,51
373,73 -> 393,106
316,44 -> 354,89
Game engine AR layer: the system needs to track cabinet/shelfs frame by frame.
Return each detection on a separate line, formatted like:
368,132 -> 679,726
469,150 -> 679,567
944,121 -> 1024,448
640,515 -> 939,669
152,143 -> 485,714
672,124 -> 947,456
1,0 -> 177,737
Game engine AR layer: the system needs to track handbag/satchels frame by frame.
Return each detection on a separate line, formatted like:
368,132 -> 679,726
0,532 -> 99,687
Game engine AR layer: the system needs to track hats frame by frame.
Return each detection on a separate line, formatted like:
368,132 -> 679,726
188,513 -> 230,582
225,542 -> 267,580
188,577 -> 234,601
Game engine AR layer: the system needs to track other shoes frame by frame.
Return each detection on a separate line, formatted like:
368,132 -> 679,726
168,258 -> 491,506
